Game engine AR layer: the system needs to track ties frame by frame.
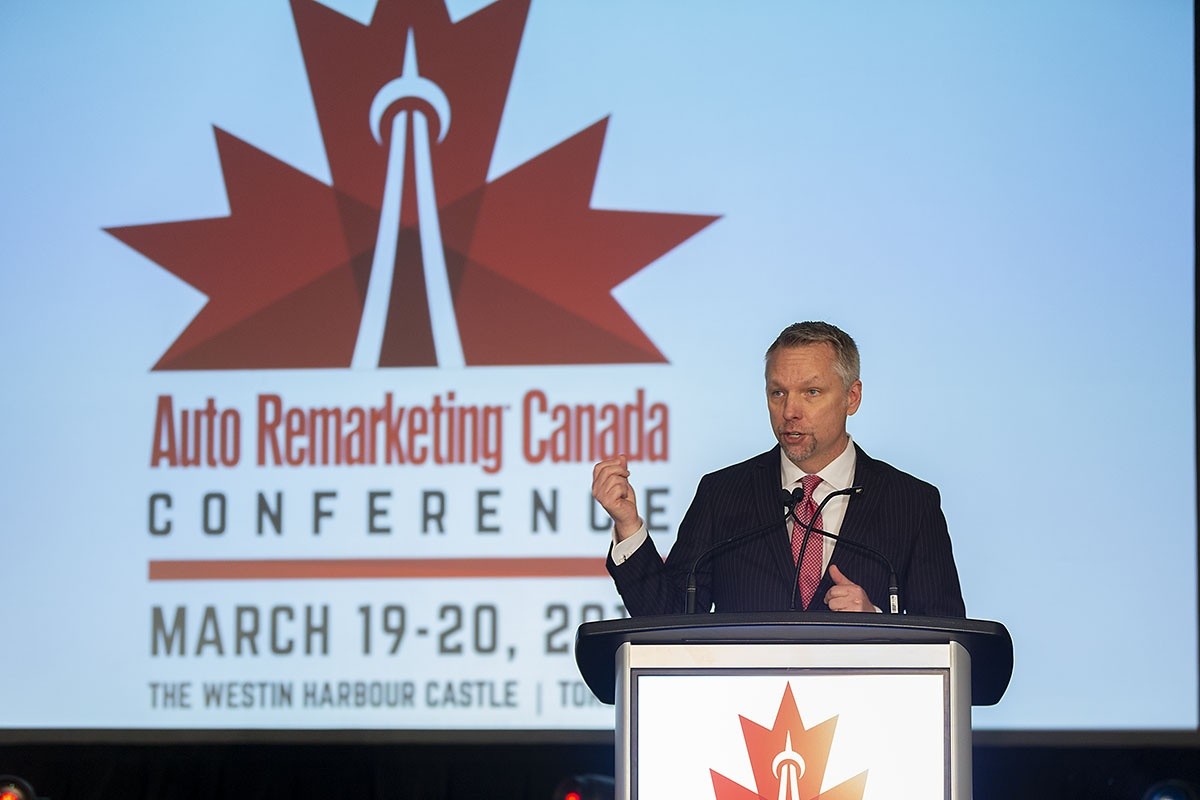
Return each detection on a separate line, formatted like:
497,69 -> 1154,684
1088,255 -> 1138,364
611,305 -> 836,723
790,474 -> 823,612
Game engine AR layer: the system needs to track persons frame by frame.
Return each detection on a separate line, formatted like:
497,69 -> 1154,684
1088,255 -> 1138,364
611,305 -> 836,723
591,321 -> 967,617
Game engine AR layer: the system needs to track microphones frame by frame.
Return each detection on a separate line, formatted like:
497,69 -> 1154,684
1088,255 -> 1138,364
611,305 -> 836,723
681,485 -> 805,613
788,485 -> 865,612
777,489 -> 903,614
